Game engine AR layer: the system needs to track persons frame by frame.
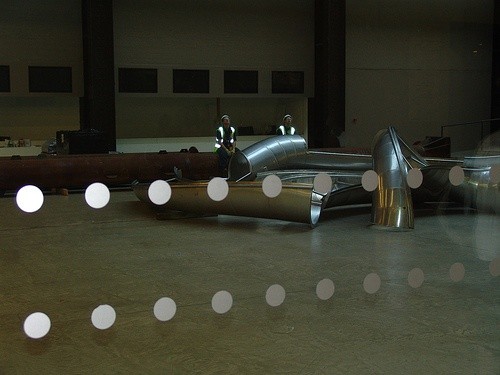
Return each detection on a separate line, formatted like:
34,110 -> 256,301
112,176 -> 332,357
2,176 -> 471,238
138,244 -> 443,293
274,114 -> 299,137
216,113 -> 240,172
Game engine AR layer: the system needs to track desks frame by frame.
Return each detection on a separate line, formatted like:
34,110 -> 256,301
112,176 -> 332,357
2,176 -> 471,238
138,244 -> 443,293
0,152 -> 216,197
